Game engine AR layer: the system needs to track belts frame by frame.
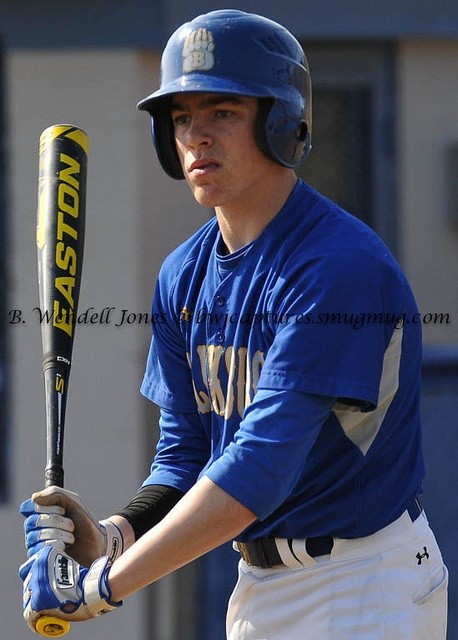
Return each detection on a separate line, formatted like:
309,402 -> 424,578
238,497 -> 423,567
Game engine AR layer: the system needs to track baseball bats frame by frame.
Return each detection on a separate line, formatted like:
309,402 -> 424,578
34,124 -> 88,636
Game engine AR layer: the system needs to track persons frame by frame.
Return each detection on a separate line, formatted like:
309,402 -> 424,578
16,7 -> 449,640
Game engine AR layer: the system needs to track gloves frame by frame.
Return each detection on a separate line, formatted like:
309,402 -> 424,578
19,485 -> 124,567
19,546 -> 123,634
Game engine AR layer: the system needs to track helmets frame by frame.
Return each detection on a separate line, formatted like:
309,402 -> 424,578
137,9 -> 312,180
